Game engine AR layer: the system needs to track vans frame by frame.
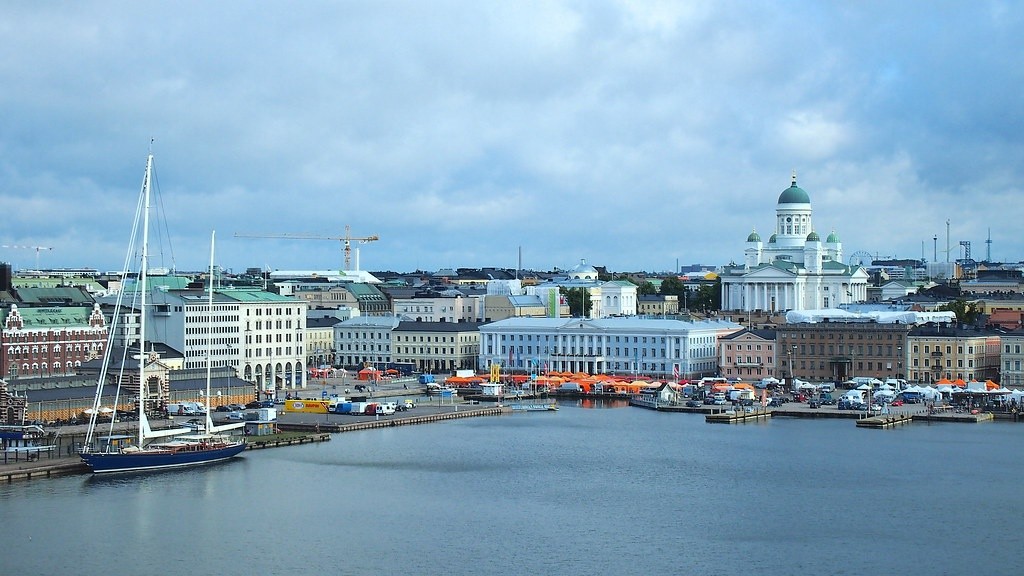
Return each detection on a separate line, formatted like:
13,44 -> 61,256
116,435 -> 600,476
335,403 -> 350,415
375,405 -> 395,416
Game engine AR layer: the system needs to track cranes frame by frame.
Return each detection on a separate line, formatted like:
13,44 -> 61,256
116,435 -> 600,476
0,244 -> 55,269
233,225 -> 379,270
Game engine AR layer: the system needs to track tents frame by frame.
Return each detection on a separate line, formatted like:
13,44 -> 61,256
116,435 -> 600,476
799,383 -> 815,388
534,372 -> 755,396
843,377 -> 1024,403
359,367 -> 398,380
446,374 -> 531,388
83,406 -> 114,418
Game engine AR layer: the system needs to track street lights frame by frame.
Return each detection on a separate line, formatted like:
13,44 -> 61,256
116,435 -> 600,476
787,349 -> 794,378
897,361 -> 900,379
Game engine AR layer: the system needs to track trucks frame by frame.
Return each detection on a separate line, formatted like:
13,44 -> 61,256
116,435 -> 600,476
555,383 -> 580,393
168,404 -> 196,416
177,401 -> 207,416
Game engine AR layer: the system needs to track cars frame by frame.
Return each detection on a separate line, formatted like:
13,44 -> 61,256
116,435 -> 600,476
384,402 -> 396,407
395,405 -> 407,412
686,378 -> 924,411
245,401 -> 262,409
607,388 -> 615,392
405,402 -> 417,409
277,398 -> 285,404
260,401 -> 274,408
350,402 -> 370,416
215,406 -> 233,412
228,403 -> 246,411
366,403 -> 380,416
328,402 -> 338,414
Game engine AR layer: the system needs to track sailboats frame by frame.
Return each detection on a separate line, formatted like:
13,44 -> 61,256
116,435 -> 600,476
78,137 -> 248,475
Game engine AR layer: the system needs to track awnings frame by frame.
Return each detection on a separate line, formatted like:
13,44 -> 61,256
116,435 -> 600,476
276,374 -> 287,378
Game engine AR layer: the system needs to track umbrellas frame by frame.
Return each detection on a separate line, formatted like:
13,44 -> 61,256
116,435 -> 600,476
71,411 -> 77,423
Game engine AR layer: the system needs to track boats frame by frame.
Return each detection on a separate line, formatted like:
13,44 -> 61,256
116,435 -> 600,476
0,425 -> 57,453
